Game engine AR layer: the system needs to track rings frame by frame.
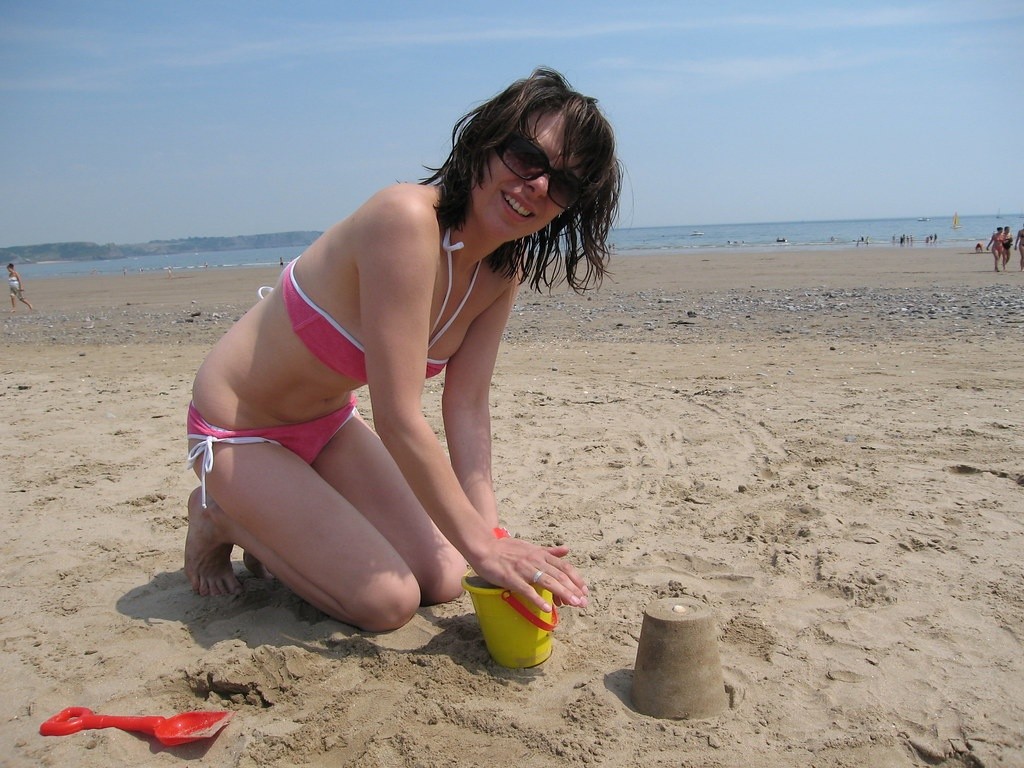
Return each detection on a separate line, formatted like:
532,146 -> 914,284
531,571 -> 543,584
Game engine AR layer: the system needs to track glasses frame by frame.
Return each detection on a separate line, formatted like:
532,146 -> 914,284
494,132 -> 586,211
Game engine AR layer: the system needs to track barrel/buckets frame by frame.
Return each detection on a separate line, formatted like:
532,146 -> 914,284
461,571 -> 558,669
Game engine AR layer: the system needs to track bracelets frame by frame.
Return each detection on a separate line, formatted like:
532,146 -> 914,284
493,527 -> 511,539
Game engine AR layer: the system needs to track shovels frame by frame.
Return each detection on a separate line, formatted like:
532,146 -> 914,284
39,706 -> 236,746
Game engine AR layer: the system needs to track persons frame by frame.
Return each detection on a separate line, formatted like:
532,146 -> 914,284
204,261 -> 208,272
280,257 -> 283,270
168,267 -> 176,280
6,263 -> 32,313
861,236 -> 869,246
893,233 -> 914,247
975,242 -> 984,252
1015,224 -> 1024,271
184,67 -> 622,633
929,234 -> 937,243
986,226 -> 1013,272
